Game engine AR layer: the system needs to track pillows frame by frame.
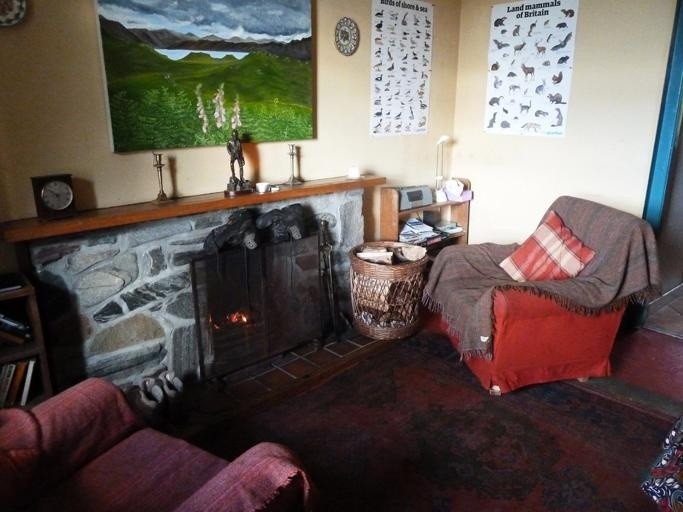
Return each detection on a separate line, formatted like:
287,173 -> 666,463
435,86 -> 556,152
496,208 -> 596,283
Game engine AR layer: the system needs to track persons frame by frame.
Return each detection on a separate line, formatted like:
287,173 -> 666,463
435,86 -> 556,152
226,128 -> 245,184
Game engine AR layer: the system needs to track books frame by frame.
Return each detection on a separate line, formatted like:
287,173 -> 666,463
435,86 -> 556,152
399,212 -> 465,248
0,277 -> 35,410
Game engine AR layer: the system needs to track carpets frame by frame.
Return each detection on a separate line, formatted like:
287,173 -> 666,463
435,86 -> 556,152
217,335 -> 680,512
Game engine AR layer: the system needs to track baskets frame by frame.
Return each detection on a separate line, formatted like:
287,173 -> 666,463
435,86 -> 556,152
347,240 -> 429,341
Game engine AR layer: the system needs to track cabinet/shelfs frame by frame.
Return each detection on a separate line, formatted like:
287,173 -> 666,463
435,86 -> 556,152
375,179 -> 473,257
0,272 -> 50,410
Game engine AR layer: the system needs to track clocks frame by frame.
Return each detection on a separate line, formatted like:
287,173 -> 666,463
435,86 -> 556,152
28,172 -> 80,225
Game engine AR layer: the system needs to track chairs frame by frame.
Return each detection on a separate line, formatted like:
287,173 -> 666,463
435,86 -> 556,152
434,195 -> 642,398
0,377 -> 315,512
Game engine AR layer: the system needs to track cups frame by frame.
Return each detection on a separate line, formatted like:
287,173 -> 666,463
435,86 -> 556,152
255,182 -> 269,193
